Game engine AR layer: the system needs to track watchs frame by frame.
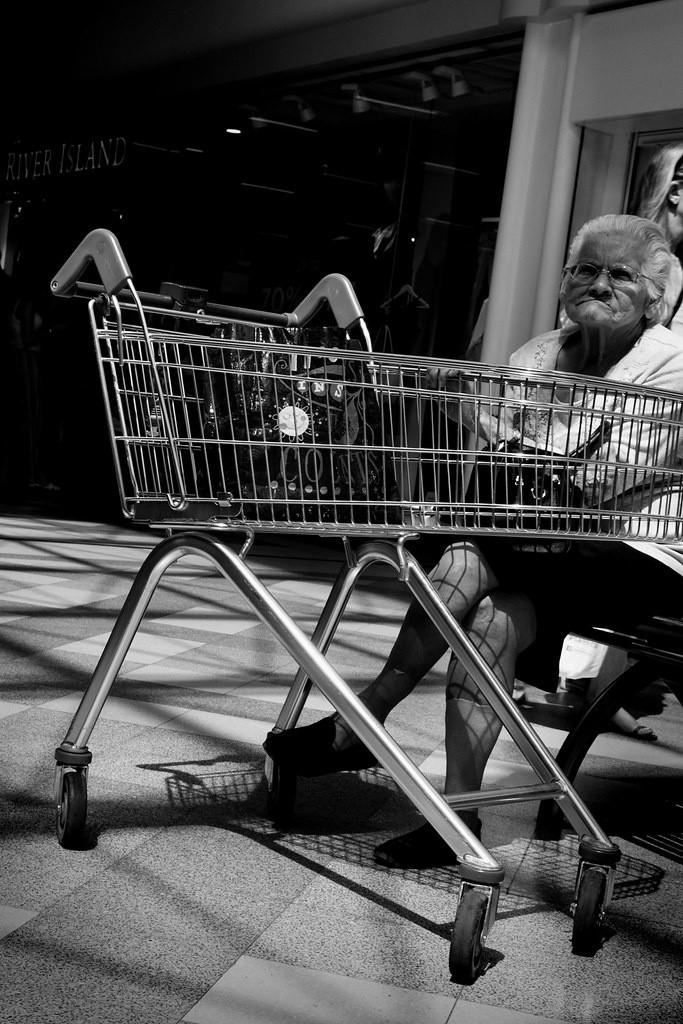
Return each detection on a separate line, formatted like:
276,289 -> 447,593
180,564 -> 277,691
499,427 -> 522,451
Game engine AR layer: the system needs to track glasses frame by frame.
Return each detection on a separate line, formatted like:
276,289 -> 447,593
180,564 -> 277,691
562,261 -> 656,289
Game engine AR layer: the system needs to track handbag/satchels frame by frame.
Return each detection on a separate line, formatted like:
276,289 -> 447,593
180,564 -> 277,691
459,421 -> 608,556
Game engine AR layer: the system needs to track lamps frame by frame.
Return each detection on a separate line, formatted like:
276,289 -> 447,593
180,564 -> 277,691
432,65 -> 470,97
242,104 -> 268,128
411,71 -> 440,102
281,94 -> 316,121
341,84 -> 370,113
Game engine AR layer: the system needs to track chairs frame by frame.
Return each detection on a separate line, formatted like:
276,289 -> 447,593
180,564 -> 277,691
535,615 -> 683,842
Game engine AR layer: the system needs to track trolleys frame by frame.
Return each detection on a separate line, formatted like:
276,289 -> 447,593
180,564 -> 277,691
49,226 -> 683,983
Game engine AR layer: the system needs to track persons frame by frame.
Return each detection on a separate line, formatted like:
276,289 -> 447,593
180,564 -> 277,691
635,141 -> 682,252
261,214 -> 683,871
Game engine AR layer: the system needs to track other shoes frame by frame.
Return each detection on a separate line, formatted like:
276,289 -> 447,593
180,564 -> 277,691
373,817 -> 481,867
262,717 -> 385,777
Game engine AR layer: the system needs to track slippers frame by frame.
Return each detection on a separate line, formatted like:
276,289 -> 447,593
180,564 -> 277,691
609,720 -> 657,741
